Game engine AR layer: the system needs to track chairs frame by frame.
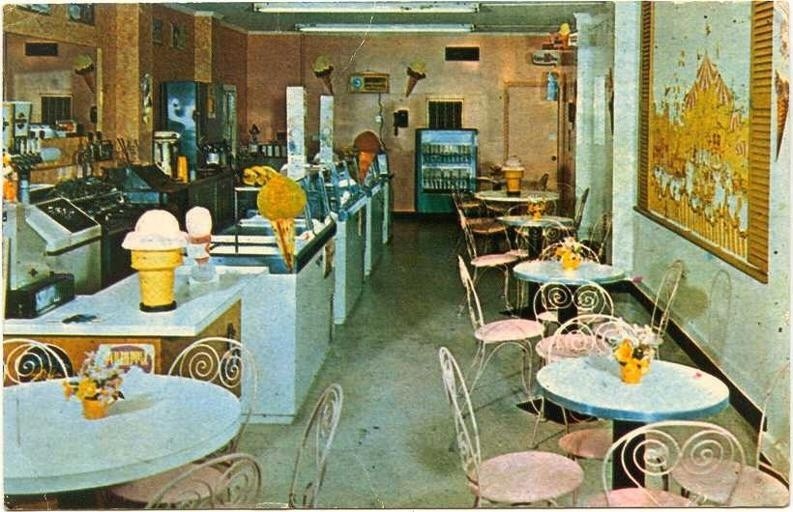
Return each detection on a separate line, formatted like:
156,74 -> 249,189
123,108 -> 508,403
258,383 -> 344,510
3,339 -> 67,388
145,453 -> 260,509
108,337 -> 258,503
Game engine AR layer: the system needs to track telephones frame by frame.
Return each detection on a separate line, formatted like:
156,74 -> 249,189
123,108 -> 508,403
393,110 -> 408,136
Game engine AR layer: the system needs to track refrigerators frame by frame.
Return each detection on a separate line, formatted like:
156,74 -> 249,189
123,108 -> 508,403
415,128 -> 478,213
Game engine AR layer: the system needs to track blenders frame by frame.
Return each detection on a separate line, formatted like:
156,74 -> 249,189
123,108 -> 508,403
153,130 -> 180,182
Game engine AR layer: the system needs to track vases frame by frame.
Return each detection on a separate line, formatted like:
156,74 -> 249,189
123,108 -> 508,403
82,399 -> 107,418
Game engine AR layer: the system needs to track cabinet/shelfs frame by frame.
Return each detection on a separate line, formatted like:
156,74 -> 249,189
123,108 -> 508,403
190,174 -> 234,231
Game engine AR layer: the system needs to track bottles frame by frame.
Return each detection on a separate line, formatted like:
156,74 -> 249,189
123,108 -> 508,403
423,169 -> 470,192
177,155 -> 188,183
190,166 -> 197,181
281,140 -> 287,158
545,72 -> 557,100
274,140 -> 281,158
261,143 -> 266,158
267,138 -> 273,157
423,145 -> 471,163
21,175 -> 30,207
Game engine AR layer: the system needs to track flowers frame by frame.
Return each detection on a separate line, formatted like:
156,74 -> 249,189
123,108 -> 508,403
61,346 -> 128,406
